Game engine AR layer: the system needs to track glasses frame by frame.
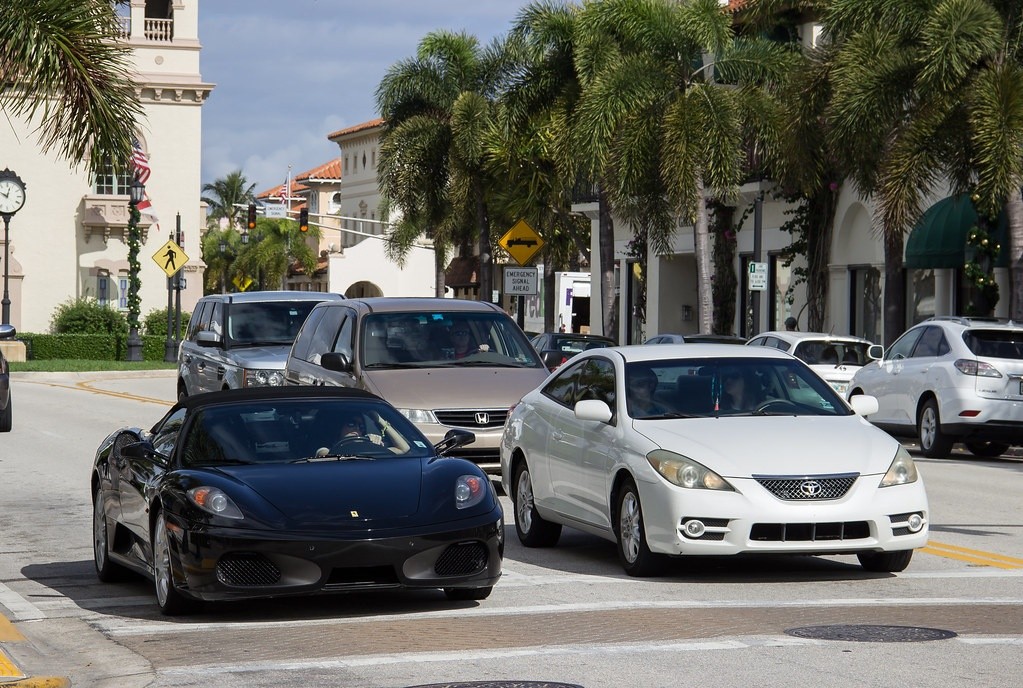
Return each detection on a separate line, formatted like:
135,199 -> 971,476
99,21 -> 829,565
347,422 -> 365,429
453,331 -> 470,336
722,373 -> 738,381
631,380 -> 648,387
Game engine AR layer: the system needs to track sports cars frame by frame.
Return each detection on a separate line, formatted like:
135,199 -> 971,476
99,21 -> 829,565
89,384 -> 507,623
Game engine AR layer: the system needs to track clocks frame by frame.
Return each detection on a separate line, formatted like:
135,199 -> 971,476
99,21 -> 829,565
0,166 -> 26,216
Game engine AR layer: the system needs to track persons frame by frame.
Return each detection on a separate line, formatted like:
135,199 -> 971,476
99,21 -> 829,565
559,324 -> 566,332
715,366 -> 779,413
305,409 -> 386,458
440,321 -> 490,359
611,367 -> 677,418
784,317 -> 797,331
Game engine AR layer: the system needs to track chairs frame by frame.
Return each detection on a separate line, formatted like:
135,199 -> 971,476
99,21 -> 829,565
820,348 -> 839,363
430,329 -> 469,359
727,373 -> 783,415
675,372 -> 713,411
205,425 -> 249,467
842,350 -> 858,365
359,334 -> 392,365
315,417 -> 367,455
625,368 -> 664,419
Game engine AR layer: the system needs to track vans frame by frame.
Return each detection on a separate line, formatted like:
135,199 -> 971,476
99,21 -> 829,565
283,296 -> 554,475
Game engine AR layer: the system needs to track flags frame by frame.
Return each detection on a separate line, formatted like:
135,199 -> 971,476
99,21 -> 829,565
281,178 -> 287,202
129,132 -> 152,212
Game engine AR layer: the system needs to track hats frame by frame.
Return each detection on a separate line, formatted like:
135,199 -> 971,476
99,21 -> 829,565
785,317 -> 797,325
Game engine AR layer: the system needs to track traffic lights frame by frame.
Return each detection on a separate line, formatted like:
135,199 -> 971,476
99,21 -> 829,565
248,204 -> 256,229
300,208 -> 308,232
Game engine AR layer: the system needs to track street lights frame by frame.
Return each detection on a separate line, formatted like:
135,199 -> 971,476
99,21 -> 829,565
218,240 -> 228,289
124,172 -> 146,362
241,231 -> 250,254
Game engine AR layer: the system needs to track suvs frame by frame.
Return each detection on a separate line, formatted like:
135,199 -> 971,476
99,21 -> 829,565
844,315 -> 1023,460
175,289 -> 348,404
744,331 -> 874,400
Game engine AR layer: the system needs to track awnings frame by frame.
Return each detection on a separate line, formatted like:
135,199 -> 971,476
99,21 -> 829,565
906,186 -> 1009,268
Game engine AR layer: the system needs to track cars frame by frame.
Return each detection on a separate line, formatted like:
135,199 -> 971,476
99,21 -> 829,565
641,334 -> 749,346
529,332 -> 621,375
0,324 -> 17,433
498,340 -> 932,585
